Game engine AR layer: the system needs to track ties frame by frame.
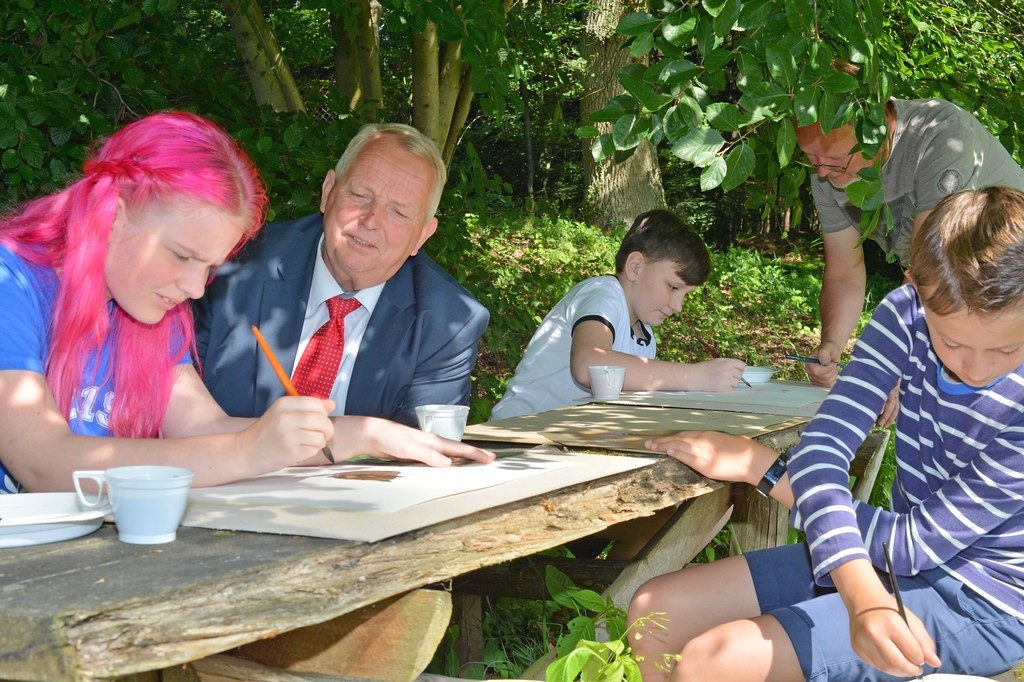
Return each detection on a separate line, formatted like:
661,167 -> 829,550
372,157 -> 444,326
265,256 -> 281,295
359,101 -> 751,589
286,296 -> 362,400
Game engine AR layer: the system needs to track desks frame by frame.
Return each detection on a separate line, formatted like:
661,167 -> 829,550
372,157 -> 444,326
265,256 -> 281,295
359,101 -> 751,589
0,376 -> 837,682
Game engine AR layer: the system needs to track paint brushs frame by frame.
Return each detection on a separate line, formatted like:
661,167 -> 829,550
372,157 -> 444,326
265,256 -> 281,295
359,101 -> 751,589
691,333 -> 752,388
785,355 -> 849,366
883,542 -> 923,682
248,318 -> 335,464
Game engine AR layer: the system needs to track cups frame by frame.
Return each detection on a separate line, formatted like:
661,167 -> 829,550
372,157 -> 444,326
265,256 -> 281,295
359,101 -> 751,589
72,466 -> 194,545
587,366 -> 627,400
416,404 -> 470,444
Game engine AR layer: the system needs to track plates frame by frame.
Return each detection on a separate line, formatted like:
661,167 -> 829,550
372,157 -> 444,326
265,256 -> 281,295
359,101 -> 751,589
0,492 -> 115,548
737,365 -> 780,382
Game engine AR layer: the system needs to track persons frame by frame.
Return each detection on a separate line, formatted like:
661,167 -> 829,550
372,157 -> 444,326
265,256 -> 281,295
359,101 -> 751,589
792,61 -> 1024,428
189,123 -> 490,431
486,209 -> 745,561
0,111 -> 495,496
626,185 -> 1024,682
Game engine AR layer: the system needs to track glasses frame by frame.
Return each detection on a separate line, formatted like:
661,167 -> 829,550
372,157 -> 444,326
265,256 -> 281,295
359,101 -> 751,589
812,153 -> 855,173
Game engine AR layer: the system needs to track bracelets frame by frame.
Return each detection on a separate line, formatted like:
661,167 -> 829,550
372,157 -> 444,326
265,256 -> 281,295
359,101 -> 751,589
755,454 -> 788,498
